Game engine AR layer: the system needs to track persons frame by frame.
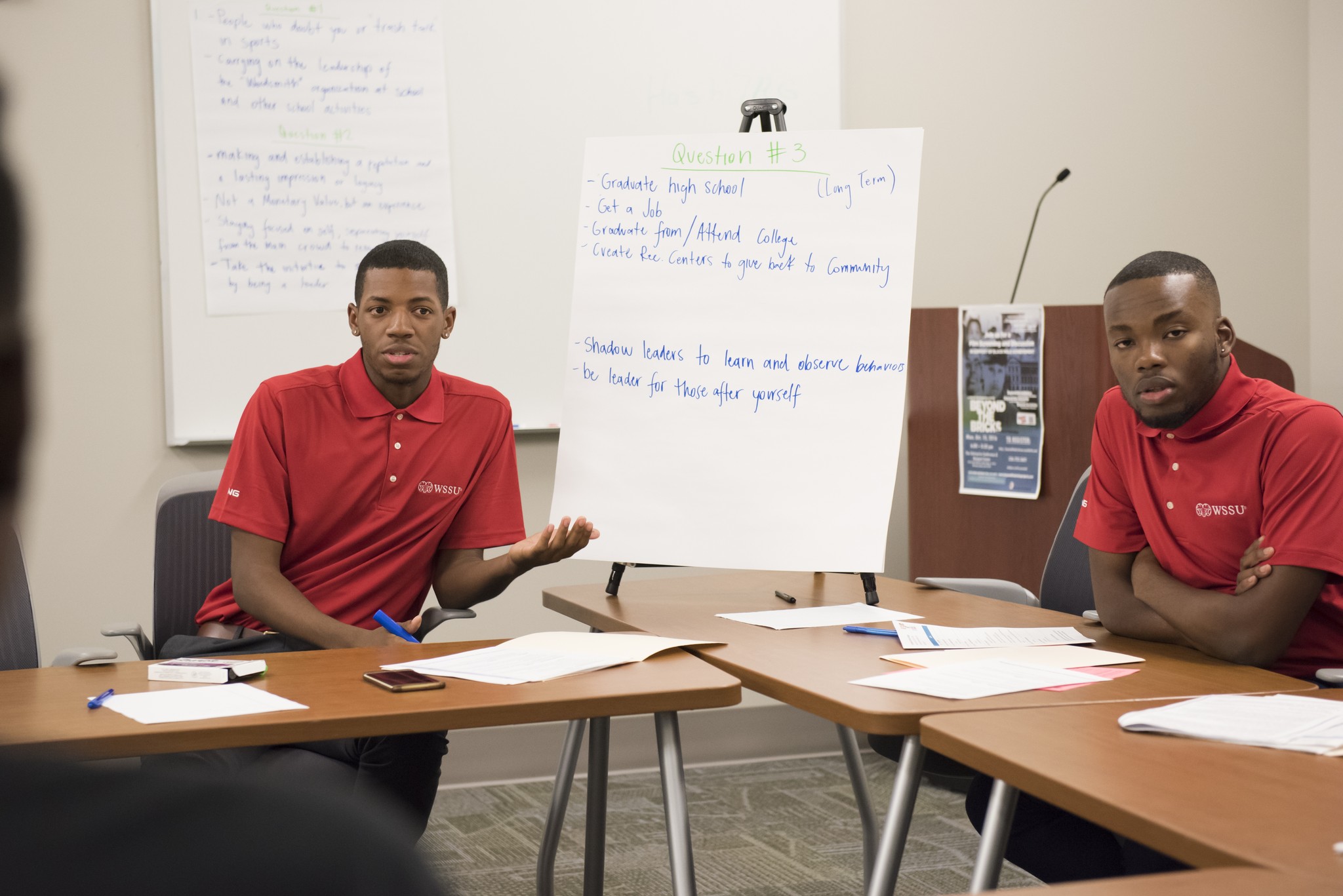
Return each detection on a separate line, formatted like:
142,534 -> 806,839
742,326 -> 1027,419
1074,251 -> 1343,688
0,112 -> 457,896
143,240 -> 600,848
963,315 -> 1040,431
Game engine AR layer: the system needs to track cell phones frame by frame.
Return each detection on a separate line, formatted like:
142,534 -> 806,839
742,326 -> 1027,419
363,668 -> 445,693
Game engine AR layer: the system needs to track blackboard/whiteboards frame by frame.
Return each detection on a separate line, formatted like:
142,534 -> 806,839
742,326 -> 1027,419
545,128 -> 924,576
147,0 -> 844,454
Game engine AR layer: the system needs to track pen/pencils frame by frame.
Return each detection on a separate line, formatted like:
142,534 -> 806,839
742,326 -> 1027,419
842,623 -> 899,636
87,689 -> 116,708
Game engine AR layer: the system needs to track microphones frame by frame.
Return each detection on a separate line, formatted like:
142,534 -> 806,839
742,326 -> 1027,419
1011,167 -> 1071,304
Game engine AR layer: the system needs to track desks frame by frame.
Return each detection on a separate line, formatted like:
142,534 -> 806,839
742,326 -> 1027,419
0,632 -> 742,896
535,575 -> 1342,895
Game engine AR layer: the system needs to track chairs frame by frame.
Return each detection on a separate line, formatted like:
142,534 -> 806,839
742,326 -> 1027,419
868,464 -> 1117,789
103,466 -> 260,674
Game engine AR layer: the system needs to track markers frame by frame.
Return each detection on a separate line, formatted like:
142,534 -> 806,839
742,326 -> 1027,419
774,590 -> 796,604
372,608 -> 423,643
